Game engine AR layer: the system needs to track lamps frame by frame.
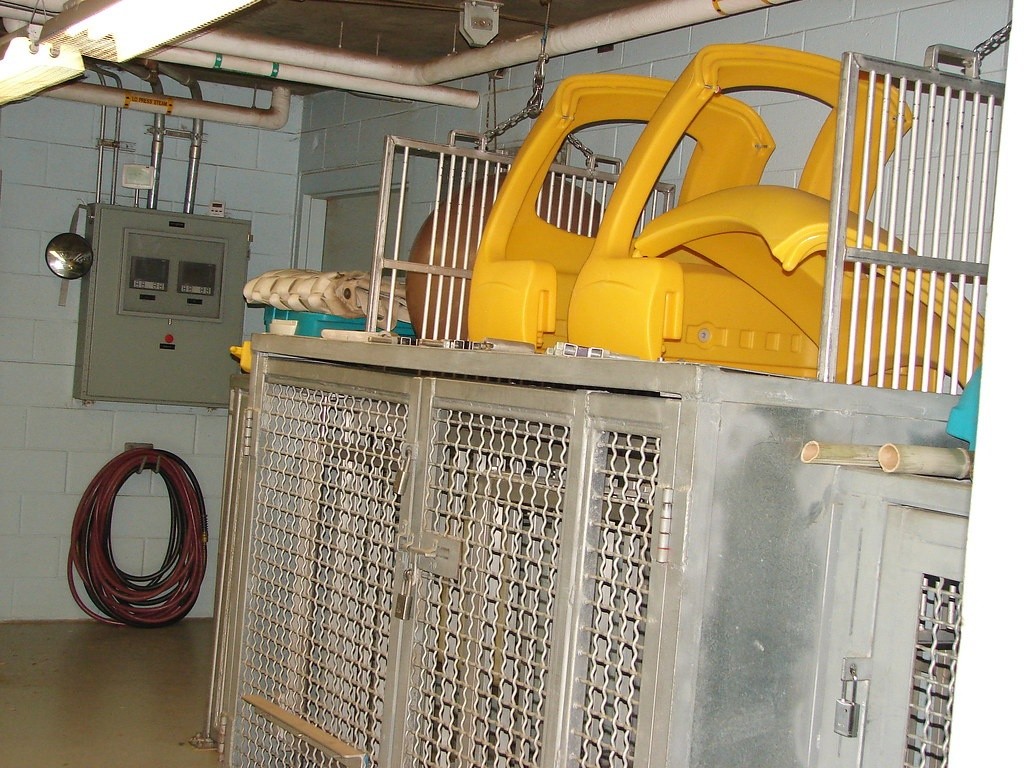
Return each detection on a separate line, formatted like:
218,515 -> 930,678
38,0 -> 265,65
0,23 -> 86,108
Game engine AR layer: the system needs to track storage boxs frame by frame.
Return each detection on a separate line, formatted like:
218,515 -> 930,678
264,305 -> 415,340
